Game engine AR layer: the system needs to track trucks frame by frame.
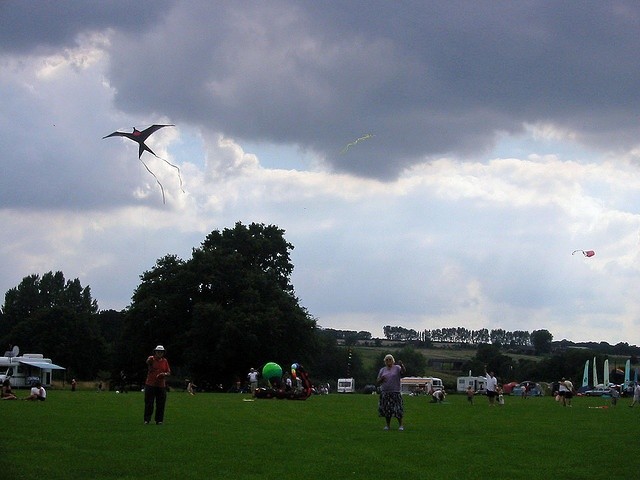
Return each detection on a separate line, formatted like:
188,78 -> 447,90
457,370 -> 488,394
400,376 -> 443,395
338,378 -> 355,394
0,354 -> 66,390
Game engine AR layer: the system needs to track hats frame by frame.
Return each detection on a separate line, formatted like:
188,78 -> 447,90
154,345 -> 166,350
250,368 -> 254,371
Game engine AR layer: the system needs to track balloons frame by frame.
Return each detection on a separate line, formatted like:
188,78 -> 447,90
254,362 -> 312,400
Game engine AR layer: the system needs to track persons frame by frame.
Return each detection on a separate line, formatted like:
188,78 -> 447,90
408,382 -> 429,396
484,365 -> 497,407
496,384 -> 504,407
285,374 -> 330,395
521,383 -> 531,400
558,377 -> 570,406
629,381 -> 640,407
247,367 -> 259,393
564,376 -> 577,406
218,384 -> 223,390
551,377 -> 560,401
429,390 -> 445,403
0,378 -> 4,398
119,370 -> 128,393
375,354 -> 406,431
19,383 -> 46,401
608,384 -> 618,409
71,378 -> 77,391
187,380 -> 197,396
97,380 -> 103,393
464,385 -> 474,407
143,345 -> 171,425
1,379 -> 17,400
234,381 -> 248,393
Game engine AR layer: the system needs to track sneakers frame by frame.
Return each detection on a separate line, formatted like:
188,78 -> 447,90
384,426 -> 390,430
399,426 -> 404,431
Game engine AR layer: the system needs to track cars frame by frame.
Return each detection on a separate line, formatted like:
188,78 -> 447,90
513,381 -> 543,396
585,387 -> 612,396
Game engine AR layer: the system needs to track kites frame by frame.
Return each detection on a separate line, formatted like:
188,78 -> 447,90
102,124 -> 185,204
342,133 -> 374,152
572,249 -> 595,257
